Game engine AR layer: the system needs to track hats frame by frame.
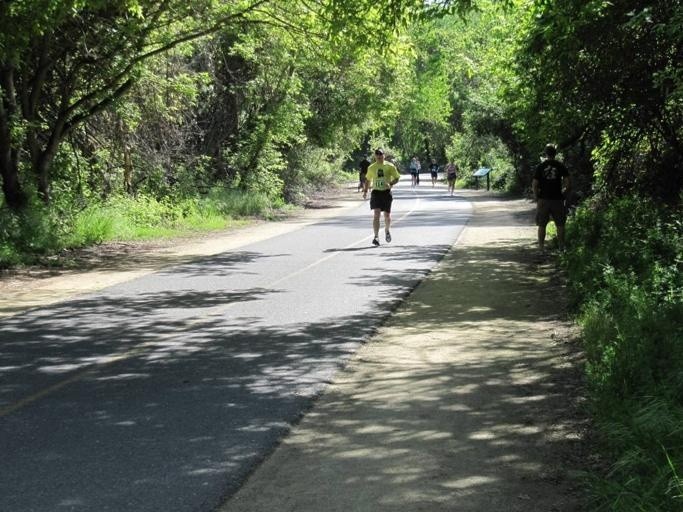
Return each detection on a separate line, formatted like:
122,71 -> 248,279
375,148 -> 384,155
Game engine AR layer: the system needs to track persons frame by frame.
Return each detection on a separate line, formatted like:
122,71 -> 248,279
415,156 -> 421,185
410,158 -> 418,188
358,159 -> 374,193
362,148 -> 400,246
444,157 -> 460,197
531,145 -> 573,252
427,158 -> 439,188
359,153 -> 372,200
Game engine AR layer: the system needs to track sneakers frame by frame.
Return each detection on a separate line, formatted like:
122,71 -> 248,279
373,233 -> 391,246
